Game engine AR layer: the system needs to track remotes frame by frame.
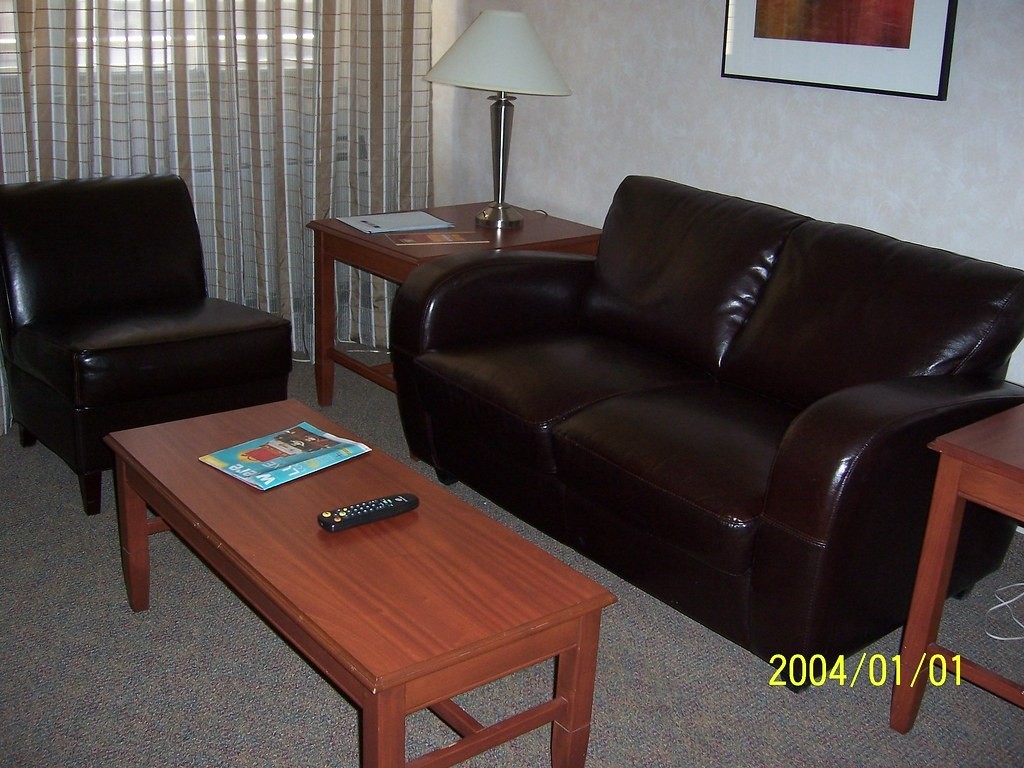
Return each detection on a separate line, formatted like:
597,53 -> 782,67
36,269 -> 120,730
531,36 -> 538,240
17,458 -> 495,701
318,493 -> 419,533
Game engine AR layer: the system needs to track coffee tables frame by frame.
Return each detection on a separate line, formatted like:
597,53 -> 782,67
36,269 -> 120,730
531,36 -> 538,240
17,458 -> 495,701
101,398 -> 618,768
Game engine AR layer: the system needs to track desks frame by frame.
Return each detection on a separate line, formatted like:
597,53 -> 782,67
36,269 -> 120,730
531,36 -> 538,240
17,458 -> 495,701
305,201 -> 606,407
889,404 -> 1024,735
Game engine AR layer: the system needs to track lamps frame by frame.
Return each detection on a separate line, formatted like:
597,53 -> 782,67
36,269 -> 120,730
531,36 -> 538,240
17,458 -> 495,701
423,11 -> 572,229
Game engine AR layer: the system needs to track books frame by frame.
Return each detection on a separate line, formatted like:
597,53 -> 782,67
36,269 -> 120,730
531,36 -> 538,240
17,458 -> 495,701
198,421 -> 373,491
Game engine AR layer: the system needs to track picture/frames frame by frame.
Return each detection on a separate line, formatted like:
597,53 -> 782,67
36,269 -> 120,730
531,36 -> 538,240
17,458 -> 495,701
722,0 -> 959,102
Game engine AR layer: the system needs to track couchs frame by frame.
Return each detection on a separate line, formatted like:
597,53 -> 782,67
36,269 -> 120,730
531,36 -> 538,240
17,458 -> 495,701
390,170 -> 1024,692
0,172 -> 294,515
